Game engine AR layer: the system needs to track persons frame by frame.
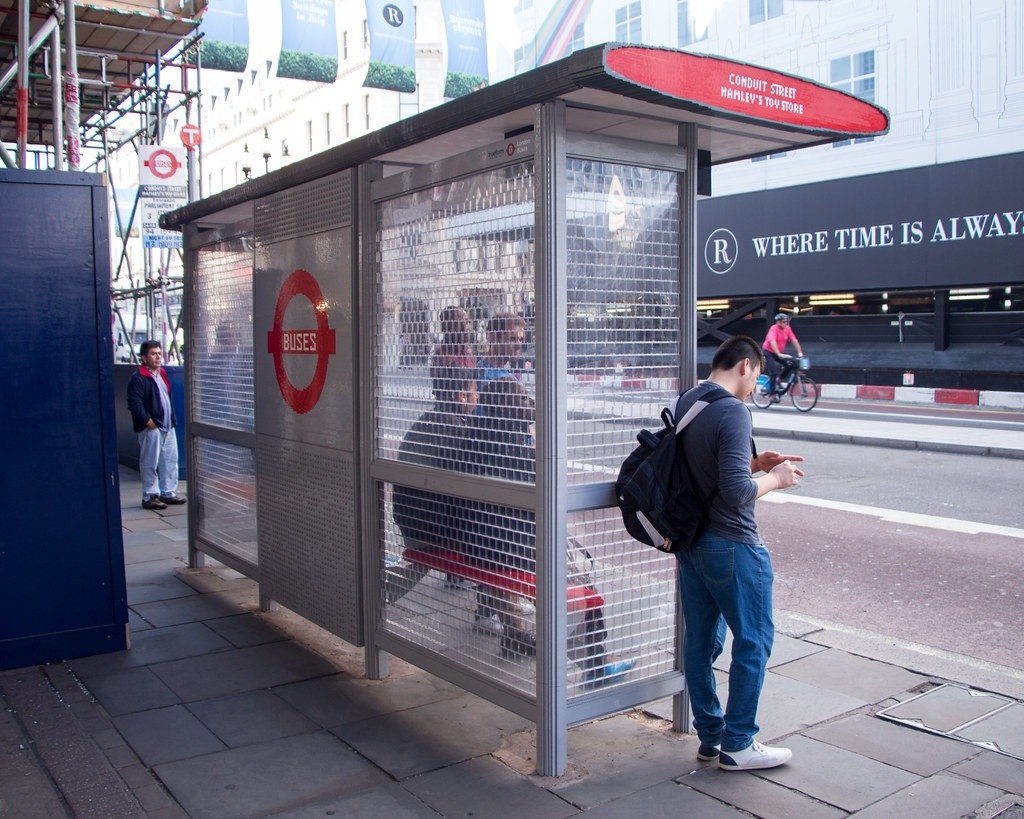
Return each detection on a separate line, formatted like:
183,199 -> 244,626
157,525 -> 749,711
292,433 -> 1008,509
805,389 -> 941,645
448,377 -> 635,689
127,341 -> 186,509
760,314 -> 802,401
426,301 -> 481,406
391,363 -> 549,660
616,335 -> 804,772
466,312 -> 542,631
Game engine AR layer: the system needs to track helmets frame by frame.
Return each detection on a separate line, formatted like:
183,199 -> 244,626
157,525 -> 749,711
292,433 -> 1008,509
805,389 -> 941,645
774,313 -> 788,320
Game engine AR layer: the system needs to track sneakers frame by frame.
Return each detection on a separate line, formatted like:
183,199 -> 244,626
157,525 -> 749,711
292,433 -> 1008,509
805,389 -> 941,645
770,392 -> 776,400
142,496 -> 167,509
440,578 -> 638,687
719,739 -> 792,770
697,741 -> 721,760
776,377 -> 783,388
160,496 -> 186,504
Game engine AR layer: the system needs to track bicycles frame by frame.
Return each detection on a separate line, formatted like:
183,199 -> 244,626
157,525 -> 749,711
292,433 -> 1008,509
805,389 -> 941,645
747,348 -> 821,412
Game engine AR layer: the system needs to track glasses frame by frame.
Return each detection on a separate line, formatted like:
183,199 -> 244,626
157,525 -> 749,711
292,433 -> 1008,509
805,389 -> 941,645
779,320 -> 788,323
498,335 -> 525,343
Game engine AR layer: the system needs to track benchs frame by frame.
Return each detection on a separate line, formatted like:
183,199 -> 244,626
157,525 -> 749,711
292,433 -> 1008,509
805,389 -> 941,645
384,540 -> 609,689
194,470 -> 259,561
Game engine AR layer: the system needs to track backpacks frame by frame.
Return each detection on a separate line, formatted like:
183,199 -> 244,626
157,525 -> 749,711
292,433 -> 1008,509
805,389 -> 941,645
614,387 -> 757,553
